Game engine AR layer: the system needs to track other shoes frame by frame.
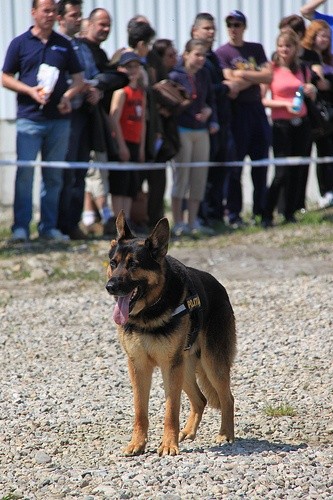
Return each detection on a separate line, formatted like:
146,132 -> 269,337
262,221 -> 272,227
46,229 -> 70,240
186,220 -> 216,235
172,224 -> 187,236
229,217 -> 249,227
317,192 -> 333,209
60,228 -> 88,240
12,227 -> 27,242
282,216 -> 298,226
211,219 -> 233,233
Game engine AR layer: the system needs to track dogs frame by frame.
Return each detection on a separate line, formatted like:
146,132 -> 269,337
104,208 -> 238,458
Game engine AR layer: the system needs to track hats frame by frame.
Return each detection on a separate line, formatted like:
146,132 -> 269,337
225,9 -> 246,24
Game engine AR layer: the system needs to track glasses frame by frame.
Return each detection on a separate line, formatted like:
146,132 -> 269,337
227,22 -> 240,28
169,52 -> 175,58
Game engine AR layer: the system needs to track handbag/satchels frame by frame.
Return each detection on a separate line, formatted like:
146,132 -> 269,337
300,61 -> 328,127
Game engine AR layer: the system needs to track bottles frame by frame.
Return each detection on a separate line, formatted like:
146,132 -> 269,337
248,57 -> 259,71
292,85 -> 304,111
236,56 -> 245,72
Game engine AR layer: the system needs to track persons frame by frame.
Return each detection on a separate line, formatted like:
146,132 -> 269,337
79,6 -> 120,237
180,14 -> 234,236
154,38 -> 214,237
260,27 -> 316,229
299,1 -> 333,30
299,19 -> 333,213
0,0 -> 85,243
54,0 -> 98,239
215,8 -> 274,233
108,14 -> 186,236
272,16 -> 331,213
